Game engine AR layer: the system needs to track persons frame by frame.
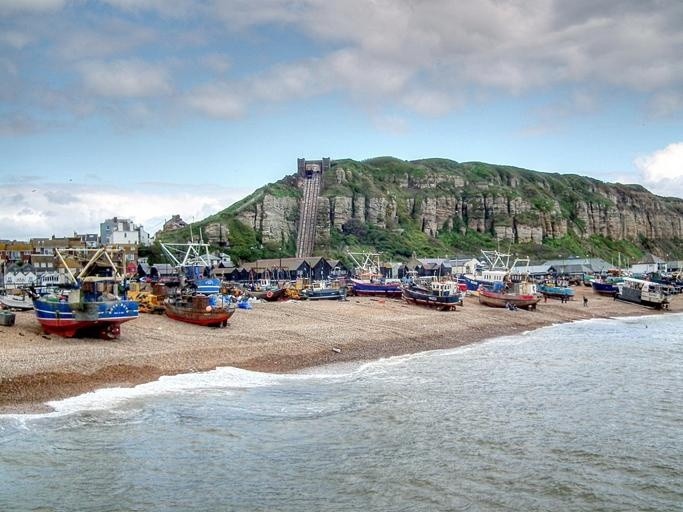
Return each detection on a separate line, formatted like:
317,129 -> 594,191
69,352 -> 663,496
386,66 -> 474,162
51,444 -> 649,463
582,296 -> 588,307
505,301 -> 517,311
542,290 -> 548,302
559,293 -> 564,303
564,292 -> 568,303
